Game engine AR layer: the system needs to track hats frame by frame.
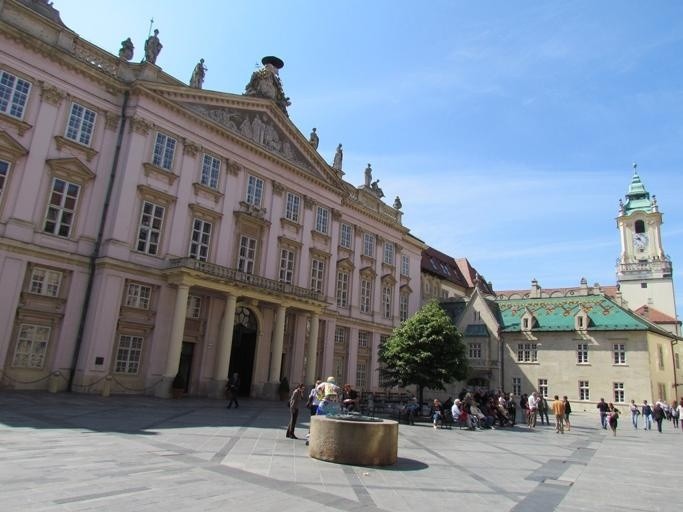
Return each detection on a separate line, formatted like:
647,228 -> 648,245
327,377 -> 335,382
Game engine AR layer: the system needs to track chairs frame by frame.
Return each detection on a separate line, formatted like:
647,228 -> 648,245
341,386 -> 518,431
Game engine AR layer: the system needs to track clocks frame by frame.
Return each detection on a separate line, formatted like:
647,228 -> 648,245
632,233 -> 647,250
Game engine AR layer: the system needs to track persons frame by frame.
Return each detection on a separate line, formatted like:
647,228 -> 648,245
629,400 -> 638,430
286,383 -> 305,439
225,372 -> 240,409
310,127 -> 319,148
606,402 -> 621,437
368,393 -> 374,414
305,376 -> 360,416
144,29 -> 162,64
432,388 -> 571,434
642,396 -> 683,432
190,58 -> 207,88
401,397 -> 419,426
333,143 -> 343,170
597,397 -> 608,430
373,178 -> 385,198
365,163 -> 373,188
